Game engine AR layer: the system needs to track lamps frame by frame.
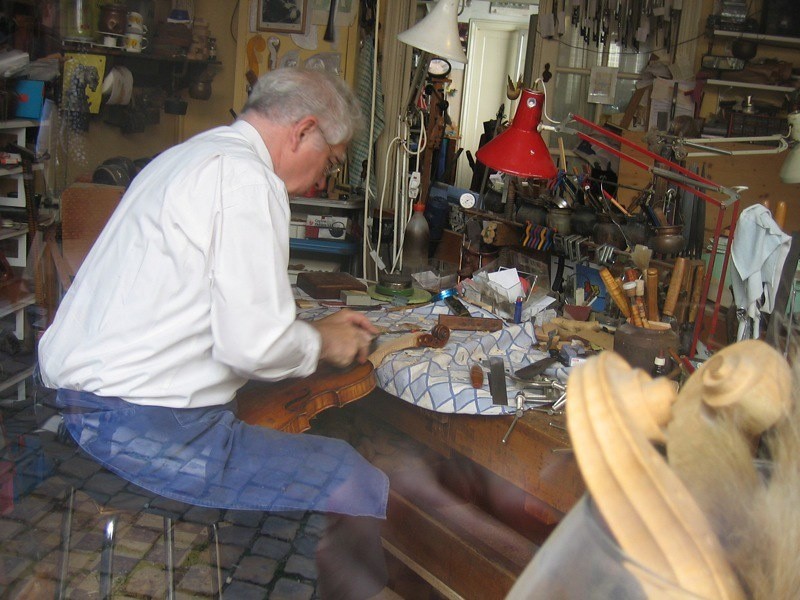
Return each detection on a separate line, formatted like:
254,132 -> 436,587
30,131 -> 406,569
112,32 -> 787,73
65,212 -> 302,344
476,77 -> 741,361
396,0 -> 468,64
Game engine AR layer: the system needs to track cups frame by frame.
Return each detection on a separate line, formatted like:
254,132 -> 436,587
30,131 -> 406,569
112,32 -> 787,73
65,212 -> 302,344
732,25 -> 759,60
103,12 -> 148,53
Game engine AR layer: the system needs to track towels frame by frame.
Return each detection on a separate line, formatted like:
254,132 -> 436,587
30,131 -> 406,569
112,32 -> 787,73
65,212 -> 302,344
730,203 -> 792,315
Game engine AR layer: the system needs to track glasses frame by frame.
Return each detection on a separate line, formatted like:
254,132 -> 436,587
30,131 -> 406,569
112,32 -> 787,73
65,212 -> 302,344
296,117 -> 343,176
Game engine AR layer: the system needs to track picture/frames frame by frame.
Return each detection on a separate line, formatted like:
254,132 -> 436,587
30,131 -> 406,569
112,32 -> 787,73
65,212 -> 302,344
587,65 -> 618,105
258,0 -> 308,34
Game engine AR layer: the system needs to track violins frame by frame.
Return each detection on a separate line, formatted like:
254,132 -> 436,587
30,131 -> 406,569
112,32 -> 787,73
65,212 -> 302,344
236,324 -> 450,434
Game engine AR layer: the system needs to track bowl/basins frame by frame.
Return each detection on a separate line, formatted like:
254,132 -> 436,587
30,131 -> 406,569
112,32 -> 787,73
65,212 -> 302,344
188,80 -> 212,100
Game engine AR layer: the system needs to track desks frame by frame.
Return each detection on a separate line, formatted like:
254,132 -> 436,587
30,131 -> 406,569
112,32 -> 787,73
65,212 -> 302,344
291,283 -> 587,600
63,45 -> 222,96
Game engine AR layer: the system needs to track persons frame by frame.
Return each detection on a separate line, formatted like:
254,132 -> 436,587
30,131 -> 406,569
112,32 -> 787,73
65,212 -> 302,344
39,66 -> 390,600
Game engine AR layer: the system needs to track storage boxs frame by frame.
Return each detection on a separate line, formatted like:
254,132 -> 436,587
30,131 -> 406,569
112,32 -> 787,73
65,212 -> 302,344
288,196 -> 363,284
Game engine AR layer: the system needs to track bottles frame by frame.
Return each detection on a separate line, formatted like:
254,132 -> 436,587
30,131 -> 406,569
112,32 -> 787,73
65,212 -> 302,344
402,204 -> 430,278
614,319 -> 679,379
99,1 -> 128,34
208,38 -> 217,60
516,199 -> 688,254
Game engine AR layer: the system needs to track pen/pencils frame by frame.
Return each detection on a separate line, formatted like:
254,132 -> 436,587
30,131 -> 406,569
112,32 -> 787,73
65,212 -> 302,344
586,290 -> 598,306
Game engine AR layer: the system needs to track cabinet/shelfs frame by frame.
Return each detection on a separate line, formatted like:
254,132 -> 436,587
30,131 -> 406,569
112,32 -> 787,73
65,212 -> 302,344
707,30 -> 800,92
0,118 -> 59,401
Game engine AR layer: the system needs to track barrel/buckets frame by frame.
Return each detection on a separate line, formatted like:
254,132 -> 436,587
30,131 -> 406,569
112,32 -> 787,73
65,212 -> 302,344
13,80 -> 45,118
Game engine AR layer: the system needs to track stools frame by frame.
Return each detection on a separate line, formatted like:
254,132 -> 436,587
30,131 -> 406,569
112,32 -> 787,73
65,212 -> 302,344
54,457 -> 223,600
